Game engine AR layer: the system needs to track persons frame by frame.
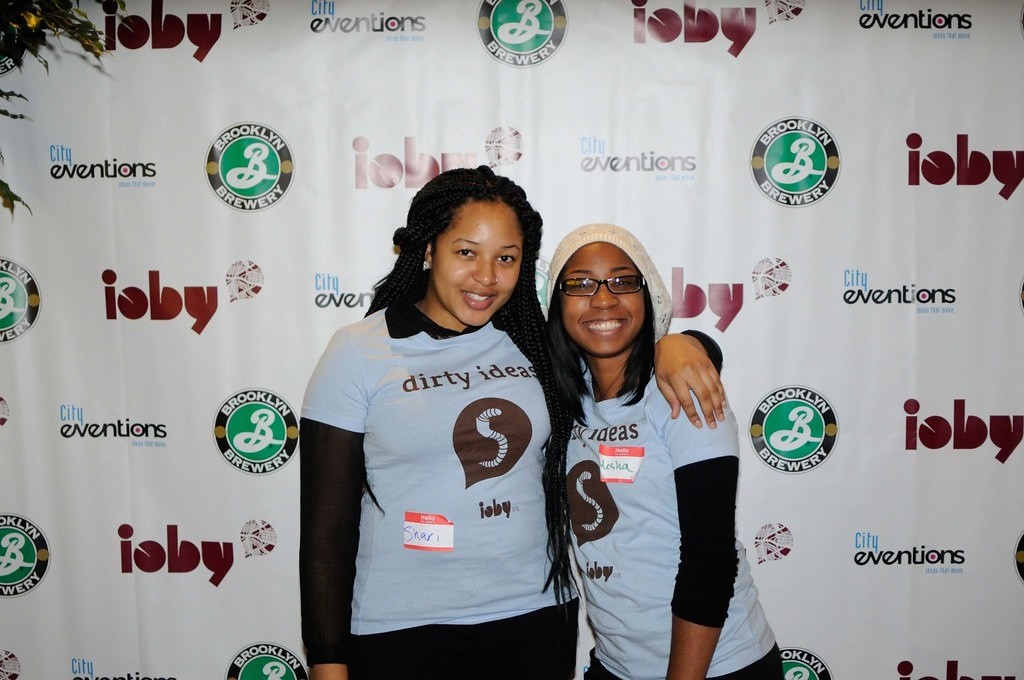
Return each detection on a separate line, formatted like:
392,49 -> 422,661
298,165 -> 726,680
545,223 -> 784,680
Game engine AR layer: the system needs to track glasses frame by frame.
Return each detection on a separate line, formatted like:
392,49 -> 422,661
558,274 -> 646,296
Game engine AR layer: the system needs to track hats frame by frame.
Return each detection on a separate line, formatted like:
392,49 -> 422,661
547,222 -> 673,345
